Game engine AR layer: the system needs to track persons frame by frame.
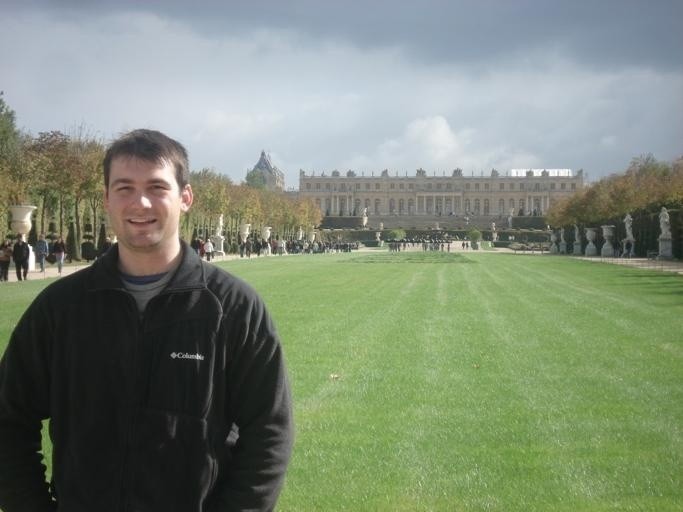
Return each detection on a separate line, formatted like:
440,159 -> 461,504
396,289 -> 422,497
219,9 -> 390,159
1,234 -> 116,281
388,231 -> 471,251
486,233 -> 515,241
0,125 -> 297,512
191,233 -> 351,261
